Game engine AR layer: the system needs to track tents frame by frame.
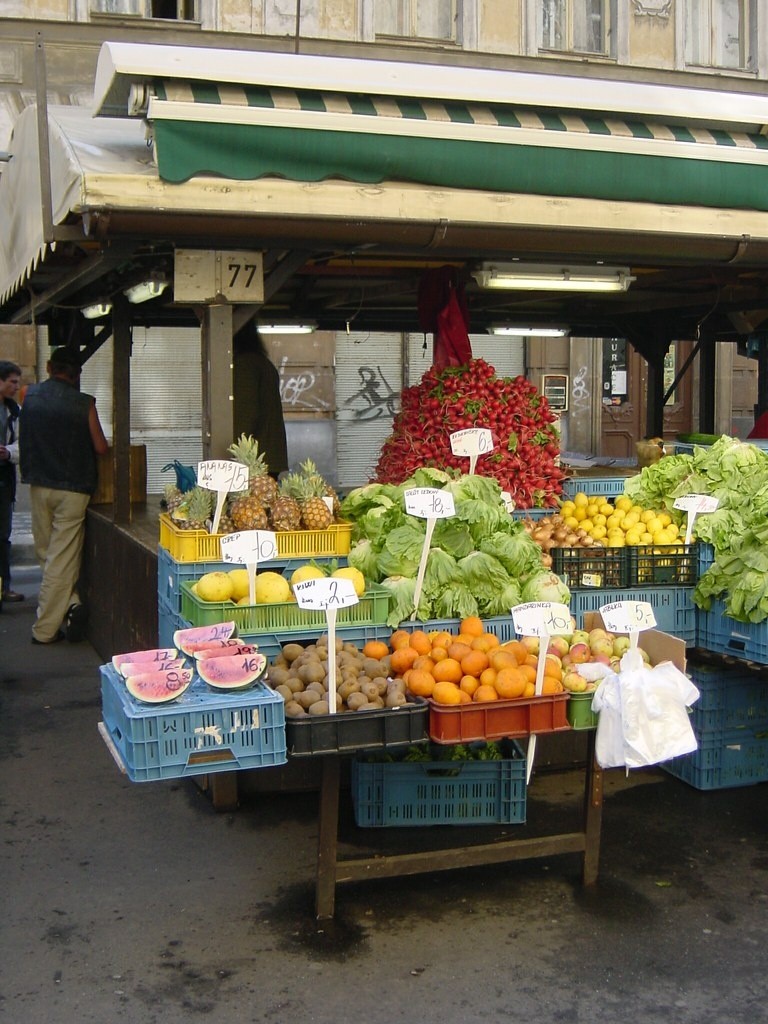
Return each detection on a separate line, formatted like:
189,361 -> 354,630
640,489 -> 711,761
0,103 -> 768,519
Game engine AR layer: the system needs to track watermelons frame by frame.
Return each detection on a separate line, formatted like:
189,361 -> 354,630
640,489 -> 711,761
111,619 -> 267,703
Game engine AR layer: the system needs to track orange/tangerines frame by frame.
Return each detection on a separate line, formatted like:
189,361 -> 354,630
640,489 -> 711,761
364,616 -> 563,705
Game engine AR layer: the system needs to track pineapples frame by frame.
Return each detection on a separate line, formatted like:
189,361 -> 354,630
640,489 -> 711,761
162,432 -> 341,535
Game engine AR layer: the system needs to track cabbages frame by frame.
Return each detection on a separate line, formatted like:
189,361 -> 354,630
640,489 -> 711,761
337,467 -> 571,628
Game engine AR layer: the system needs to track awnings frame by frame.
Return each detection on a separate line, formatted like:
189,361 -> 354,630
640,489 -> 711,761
150,80 -> 768,213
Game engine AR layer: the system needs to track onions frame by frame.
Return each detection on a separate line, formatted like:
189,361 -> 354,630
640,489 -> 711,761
519,510 -> 617,587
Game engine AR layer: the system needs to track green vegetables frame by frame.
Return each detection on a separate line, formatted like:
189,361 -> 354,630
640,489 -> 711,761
623,435 -> 768,624
363,738 -> 506,778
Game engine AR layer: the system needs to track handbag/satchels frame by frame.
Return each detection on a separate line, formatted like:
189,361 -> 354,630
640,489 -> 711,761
161,458 -> 197,494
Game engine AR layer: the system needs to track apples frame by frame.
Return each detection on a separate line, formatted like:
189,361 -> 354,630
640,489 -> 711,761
520,616 -> 654,692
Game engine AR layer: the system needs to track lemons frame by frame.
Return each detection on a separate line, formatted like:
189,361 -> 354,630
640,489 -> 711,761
558,492 -> 687,580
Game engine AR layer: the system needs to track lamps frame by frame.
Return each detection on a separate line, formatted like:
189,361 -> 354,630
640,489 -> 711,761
487,319 -> 572,339
471,261 -> 638,293
80,295 -> 113,319
124,272 -> 170,304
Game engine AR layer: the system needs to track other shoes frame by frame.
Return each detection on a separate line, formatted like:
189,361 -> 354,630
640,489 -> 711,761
65,603 -> 86,634
4,591 -> 24,602
32,630 -> 64,644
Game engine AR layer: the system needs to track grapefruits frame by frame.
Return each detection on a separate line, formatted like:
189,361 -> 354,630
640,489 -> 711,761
192,565 -> 365,607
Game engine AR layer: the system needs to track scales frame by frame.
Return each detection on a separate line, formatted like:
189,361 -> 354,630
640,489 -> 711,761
538,373 -> 597,477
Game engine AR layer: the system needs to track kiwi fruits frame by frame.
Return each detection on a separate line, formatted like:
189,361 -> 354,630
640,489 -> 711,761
266,634 -> 415,718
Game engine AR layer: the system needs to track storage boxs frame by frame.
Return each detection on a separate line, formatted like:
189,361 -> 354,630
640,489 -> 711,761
429,689 -> 571,745
98,650 -> 289,783
657,732 -> 768,790
568,588 -> 696,649
180,581 -> 392,666
159,513 -> 354,563
561,478 -> 630,507
698,539 -> 715,581
397,616 -> 522,645
511,509 -> 561,523
677,444 -> 768,458
570,673 -> 694,731
157,543 -> 346,613
697,590 -> 768,663
158,601 -> 180,649
550,544 -> 627,589
352,740 -> 528,828
286,693 -> 429,760
687,666 -> 768,733
627,542 -> 698,587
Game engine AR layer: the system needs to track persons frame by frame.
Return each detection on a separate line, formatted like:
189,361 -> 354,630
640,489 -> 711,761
0,347 -> 112,646
230,321 -> 288,480
